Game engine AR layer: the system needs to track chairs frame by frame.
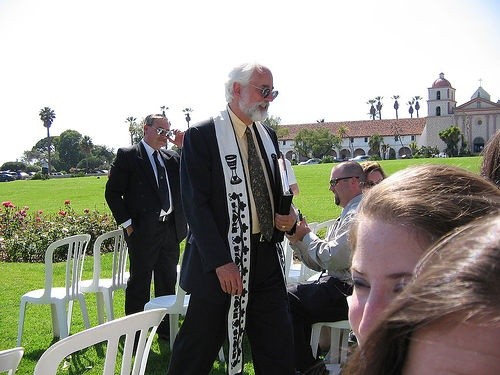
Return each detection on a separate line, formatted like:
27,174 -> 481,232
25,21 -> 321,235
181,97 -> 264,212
33,308 -> 168,375
289,218 -> 336,276
310,321 -> 353,375
15,233 -> 91,348
67,229 -> 130,336
143,285 -> 191,354
284,222 -> 316,290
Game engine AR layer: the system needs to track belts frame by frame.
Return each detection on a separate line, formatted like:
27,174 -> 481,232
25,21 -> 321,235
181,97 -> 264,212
251,234 -> 269,246
158,212 -> 174,222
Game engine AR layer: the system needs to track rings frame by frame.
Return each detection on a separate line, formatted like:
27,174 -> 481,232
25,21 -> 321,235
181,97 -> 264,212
282,225 -> 286,229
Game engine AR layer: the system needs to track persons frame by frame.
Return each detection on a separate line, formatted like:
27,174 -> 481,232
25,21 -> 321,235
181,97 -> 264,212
40,154 -> 49,180
286,161 -> 364,375
105,114 -> 188,349
340,216 -> 500,375
168,62 -> 297,375
482,131 -> 500,188
362,162 -> 384,193
292,152 -> 296,164
347,164 -> 500,350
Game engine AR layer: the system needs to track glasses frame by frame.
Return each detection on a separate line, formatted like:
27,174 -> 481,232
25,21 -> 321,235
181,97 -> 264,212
330,177 -> 359,187
150,126 -> 172,137
249,83 -> 278,99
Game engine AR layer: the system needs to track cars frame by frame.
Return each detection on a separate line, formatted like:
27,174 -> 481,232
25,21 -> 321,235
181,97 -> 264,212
51,172 -> 64,176
436,152 -> 448,158
348,156 -> 369,162
0,171 -> 36,182
300,159 -> 321,165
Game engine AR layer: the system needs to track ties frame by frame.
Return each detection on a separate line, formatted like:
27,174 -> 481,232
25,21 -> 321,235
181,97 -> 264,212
152,150 -> 170,213
244,127 -> 273,241
